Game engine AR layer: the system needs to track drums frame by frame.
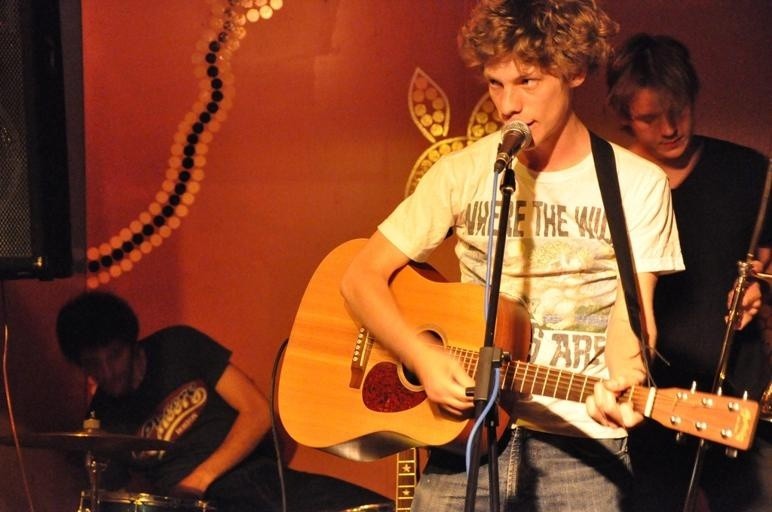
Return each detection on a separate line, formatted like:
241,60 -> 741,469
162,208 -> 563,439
76,490 -> 215,512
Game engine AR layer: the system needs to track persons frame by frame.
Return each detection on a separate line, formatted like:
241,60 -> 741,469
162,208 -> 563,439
603,31 -> 772,511
338,1 -> 692,511
55,289 -> 395,512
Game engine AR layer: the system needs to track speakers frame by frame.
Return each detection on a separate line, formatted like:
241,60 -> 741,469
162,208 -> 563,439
0,0 -> 87,282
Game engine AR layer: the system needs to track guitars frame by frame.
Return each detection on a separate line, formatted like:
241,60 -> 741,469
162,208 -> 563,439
278,239 -> 761,462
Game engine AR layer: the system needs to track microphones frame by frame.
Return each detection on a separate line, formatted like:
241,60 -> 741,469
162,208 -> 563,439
494,120 -> 532,174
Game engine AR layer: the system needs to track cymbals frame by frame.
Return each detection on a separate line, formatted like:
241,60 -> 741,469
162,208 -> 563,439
1,431 -> 175,450
